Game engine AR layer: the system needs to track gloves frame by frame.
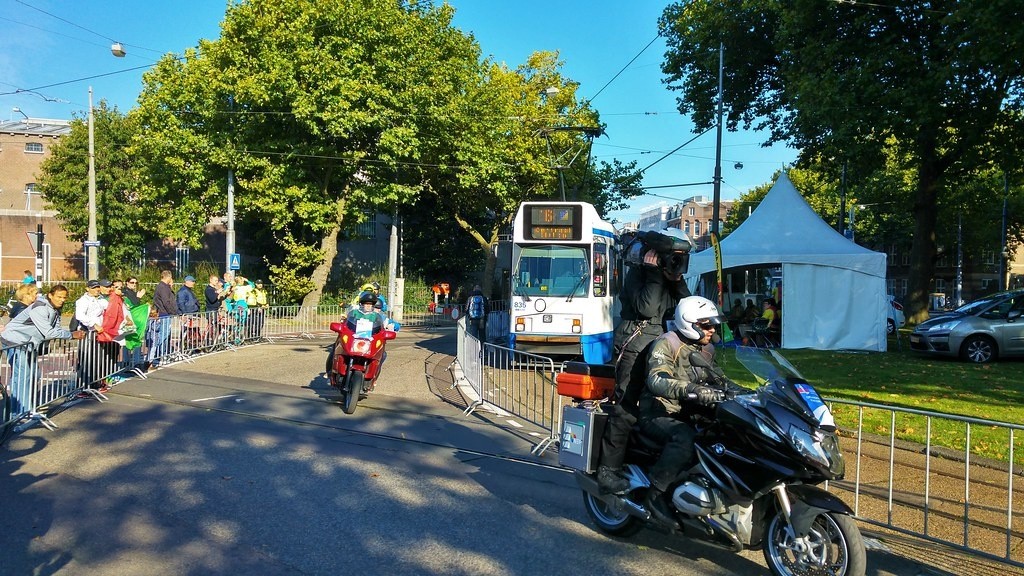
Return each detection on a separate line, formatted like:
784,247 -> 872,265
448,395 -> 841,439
687,382 -> 717,407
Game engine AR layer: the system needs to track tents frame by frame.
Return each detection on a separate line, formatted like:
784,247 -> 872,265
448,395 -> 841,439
681,174 -> 888,354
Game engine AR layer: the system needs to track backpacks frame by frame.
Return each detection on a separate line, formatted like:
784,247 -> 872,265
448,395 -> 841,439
468,296 -> 485,318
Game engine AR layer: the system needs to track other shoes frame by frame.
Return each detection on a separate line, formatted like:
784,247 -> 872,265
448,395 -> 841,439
646,488 -> 681,530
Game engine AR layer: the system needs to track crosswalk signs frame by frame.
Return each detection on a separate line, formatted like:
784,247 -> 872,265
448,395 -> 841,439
230,254 -> 241,269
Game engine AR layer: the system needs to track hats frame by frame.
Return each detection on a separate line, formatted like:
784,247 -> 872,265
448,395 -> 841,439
100,279 -> 111,287
186,276 -> 196,281
88,280 -> 100,288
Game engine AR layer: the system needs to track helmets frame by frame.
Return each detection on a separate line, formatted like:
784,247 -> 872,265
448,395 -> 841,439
675,296 -> 728,340
362,284 -> 375,292
370,281 -> 380,290
359,292 -> 377,302
374,298 -> 383,308
658,227 -> 691,247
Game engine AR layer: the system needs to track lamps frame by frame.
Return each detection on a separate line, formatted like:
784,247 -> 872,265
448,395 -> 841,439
110,42 -> 126,57
546,86 -> 560,97
13,107 -> 19,112
735,162 -> 743,171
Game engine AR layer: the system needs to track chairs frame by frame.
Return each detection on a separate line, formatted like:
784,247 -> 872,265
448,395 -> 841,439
745,316 -> 780,348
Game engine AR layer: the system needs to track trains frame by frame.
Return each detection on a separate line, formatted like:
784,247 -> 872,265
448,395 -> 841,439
507,127 -> 627,371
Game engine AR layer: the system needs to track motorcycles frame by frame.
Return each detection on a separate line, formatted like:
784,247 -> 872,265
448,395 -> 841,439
326,306 -> 396,415
557,345 -> 866,576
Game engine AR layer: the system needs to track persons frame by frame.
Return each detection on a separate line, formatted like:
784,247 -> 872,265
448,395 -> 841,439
466,285 -> 489,358
323,282 -> 401,393
0,269 -> 267,429
597,227 -> 783,533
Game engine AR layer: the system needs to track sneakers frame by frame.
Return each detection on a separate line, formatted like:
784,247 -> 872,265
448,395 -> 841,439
598,470 -> 630,495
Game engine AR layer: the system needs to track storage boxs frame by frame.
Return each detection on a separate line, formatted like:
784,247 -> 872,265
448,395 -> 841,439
555,361 -> 617,400
558,404 -> 609,475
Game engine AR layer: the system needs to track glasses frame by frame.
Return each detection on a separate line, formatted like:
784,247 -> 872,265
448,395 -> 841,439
128,281 -> 139,284
697,322 -> 712,330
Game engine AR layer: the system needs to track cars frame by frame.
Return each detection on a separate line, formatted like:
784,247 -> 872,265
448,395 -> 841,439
886,294 -> 906,336
907,286 -> 1024,364
